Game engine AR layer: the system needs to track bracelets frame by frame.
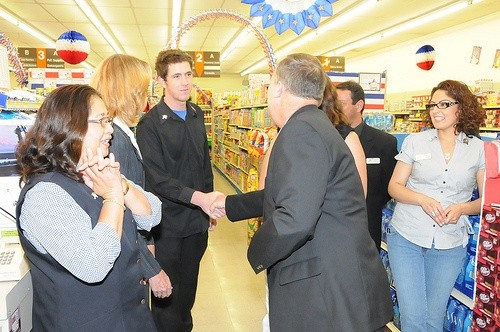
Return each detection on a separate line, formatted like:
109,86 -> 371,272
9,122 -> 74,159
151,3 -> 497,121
122,178 -> 130,195
102,199 -> 127,210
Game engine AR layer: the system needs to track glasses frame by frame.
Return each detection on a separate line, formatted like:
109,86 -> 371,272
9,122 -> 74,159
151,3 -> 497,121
88,116 -> 114,127
426,102 -> 459,110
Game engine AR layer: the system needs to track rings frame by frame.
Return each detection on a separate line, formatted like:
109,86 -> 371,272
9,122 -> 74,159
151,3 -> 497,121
431,212 -> 435,216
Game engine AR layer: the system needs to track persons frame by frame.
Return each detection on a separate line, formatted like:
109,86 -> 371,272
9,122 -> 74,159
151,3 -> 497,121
15,84 -> 163,332
387,79 -> 485,332
92,49 -> 401,332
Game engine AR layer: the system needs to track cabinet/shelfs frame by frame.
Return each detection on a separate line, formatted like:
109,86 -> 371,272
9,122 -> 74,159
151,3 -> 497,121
0,86 -> 57,121
129,78 -> 500,332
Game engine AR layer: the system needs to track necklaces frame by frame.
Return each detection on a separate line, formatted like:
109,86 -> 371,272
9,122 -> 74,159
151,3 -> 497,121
444,151 -> 451,158
